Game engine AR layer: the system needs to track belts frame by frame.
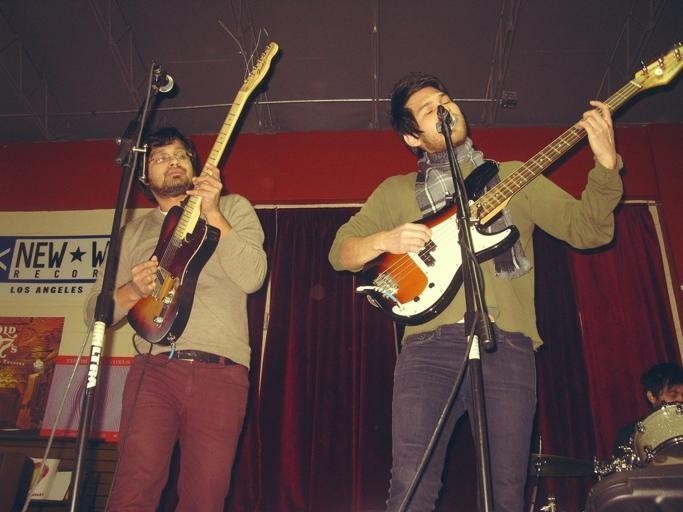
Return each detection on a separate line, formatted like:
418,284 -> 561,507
163,349 -> 234,365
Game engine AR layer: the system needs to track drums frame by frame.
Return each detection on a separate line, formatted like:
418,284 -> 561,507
632,400 -> 683,467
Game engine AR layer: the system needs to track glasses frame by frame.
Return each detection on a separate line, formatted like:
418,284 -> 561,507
148,150 -> 191,163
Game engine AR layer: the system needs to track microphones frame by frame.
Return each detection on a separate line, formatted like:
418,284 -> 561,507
153,64 -> 174,93
437,105 -> 454,127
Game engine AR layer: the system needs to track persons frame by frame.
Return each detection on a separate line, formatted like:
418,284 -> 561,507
612,358 -> 683,460
85,127 -> 267,512
326,68 -> 624,511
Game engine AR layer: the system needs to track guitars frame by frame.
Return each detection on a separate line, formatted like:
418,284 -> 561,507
128,42 -> 279,345
360,42 -> 683,326
